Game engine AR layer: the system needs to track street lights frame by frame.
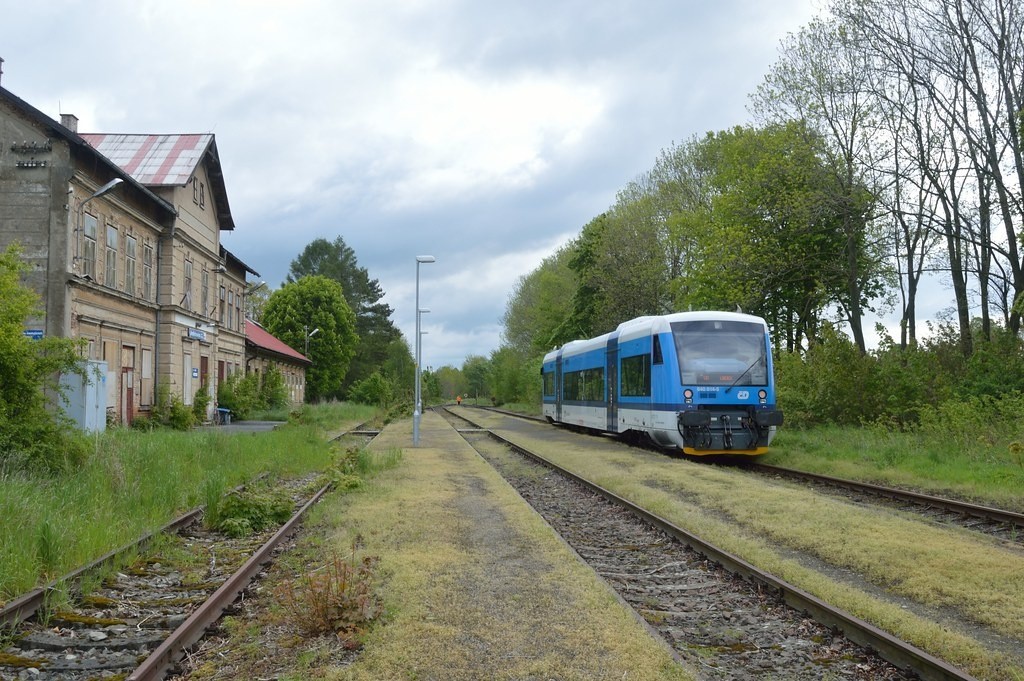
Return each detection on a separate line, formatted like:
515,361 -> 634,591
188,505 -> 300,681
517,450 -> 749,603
413,256 -> 436,447
418,309 -> 431,425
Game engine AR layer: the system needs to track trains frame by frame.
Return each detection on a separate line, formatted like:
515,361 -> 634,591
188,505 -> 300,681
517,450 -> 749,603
540,310 -> 783,457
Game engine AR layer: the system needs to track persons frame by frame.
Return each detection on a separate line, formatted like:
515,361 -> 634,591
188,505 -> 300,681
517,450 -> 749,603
457,394 -> 462,405
